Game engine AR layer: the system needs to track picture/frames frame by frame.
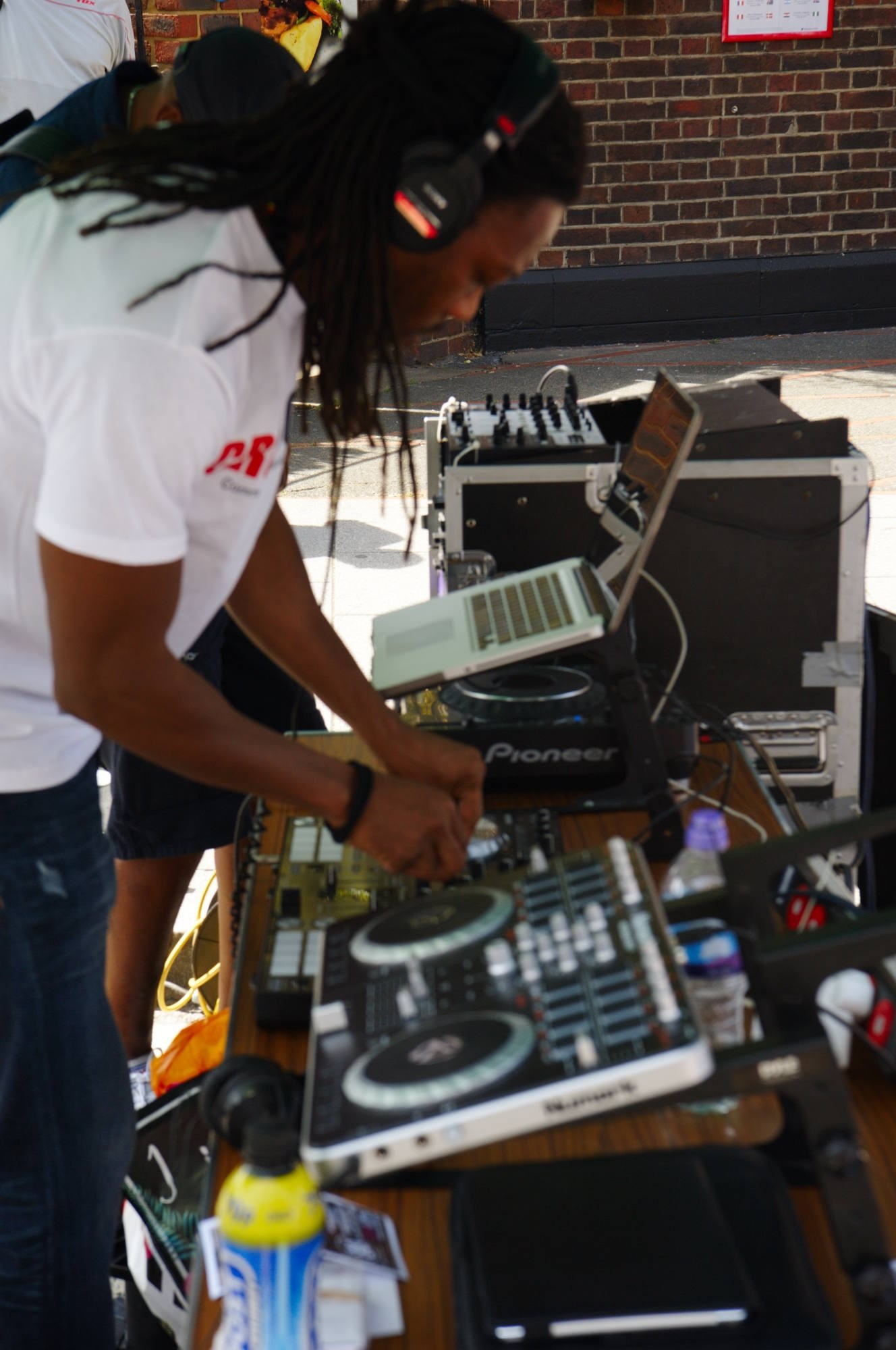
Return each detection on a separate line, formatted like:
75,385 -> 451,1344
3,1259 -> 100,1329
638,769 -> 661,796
722,0 -> 834,43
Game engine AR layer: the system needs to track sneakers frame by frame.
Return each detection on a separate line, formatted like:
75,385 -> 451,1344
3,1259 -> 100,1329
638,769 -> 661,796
130,1054 -> 157,1110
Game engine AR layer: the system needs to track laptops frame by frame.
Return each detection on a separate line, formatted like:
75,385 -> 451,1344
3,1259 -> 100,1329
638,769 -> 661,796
372,368 -> 701,698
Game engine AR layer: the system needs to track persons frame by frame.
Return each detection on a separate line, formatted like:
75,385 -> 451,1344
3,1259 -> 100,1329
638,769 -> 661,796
0,0 -> 135,123
0,0 -> 588,1350
106,27 -> 328,1117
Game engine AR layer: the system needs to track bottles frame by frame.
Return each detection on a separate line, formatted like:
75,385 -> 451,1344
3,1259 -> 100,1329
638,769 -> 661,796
211,1121 -> 327,1350
660,810 -> 750,1048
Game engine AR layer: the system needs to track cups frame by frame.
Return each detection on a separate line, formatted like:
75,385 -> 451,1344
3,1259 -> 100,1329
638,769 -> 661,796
448,549 -> 497,593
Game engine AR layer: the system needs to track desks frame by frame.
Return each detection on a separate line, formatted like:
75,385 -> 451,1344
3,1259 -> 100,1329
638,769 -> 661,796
184,729 -> 864,1350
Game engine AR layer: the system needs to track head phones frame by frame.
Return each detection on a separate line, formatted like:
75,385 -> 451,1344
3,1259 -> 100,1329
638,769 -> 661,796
196,1054 -> 305,1177
385,49 -> 559,253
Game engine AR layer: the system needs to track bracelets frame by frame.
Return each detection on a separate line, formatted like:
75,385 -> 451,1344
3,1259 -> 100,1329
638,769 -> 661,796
326,760 -> 374,841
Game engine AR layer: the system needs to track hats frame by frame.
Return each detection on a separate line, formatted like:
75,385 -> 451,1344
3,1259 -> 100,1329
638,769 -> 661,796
175,27 -> 307,125
